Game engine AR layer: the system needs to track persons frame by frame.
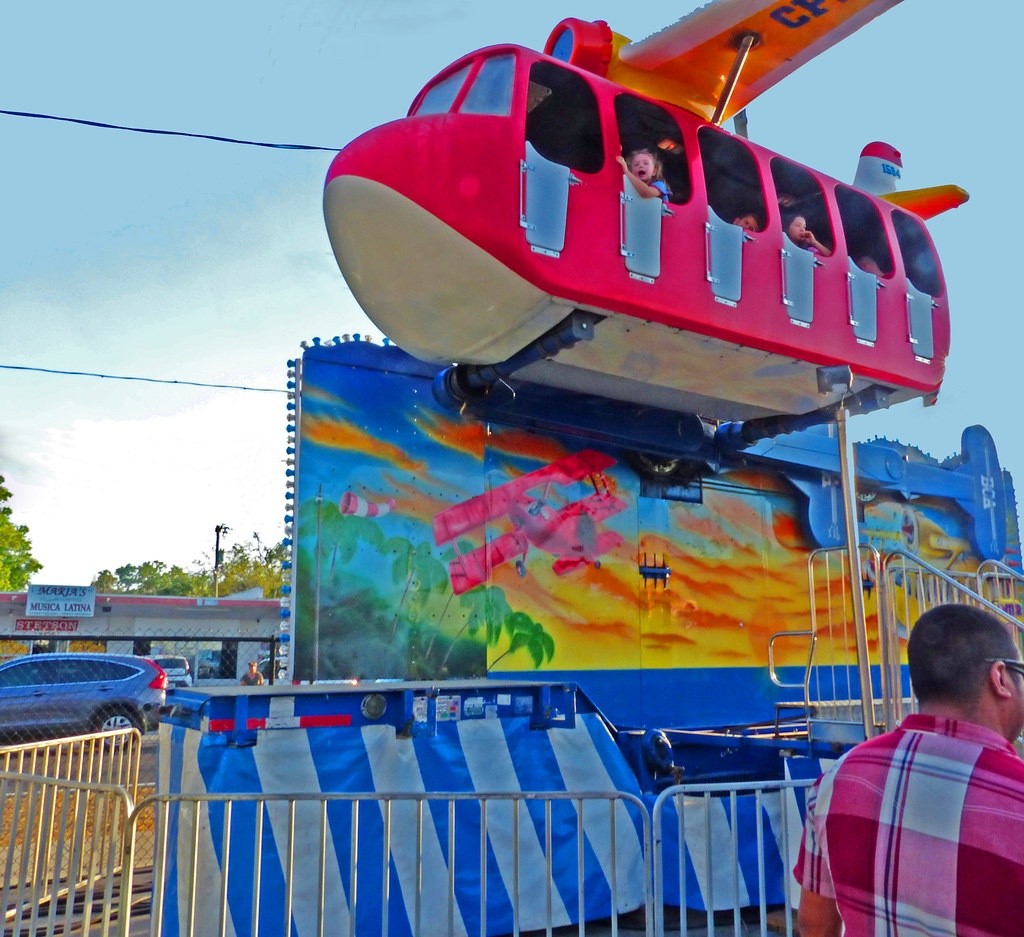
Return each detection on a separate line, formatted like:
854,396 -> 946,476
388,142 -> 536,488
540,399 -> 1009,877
240,660 -> 263,686
732,212 -> 761,232
783,213 -> 832,257
615,149 -> 673,202
795,602 -> 1023,936
856,255 -> 893,279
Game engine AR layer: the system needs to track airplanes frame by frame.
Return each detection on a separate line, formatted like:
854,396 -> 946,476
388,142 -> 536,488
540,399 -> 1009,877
321,0 -> 972,427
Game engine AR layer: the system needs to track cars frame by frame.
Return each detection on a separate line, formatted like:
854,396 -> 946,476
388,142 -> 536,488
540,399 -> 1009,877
256,655 -> 289,679
141,654 -> 192,689
187,649 -> 223,680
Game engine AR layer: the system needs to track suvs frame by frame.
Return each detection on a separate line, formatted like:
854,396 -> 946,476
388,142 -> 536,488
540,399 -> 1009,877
0,652 -> 168,752
